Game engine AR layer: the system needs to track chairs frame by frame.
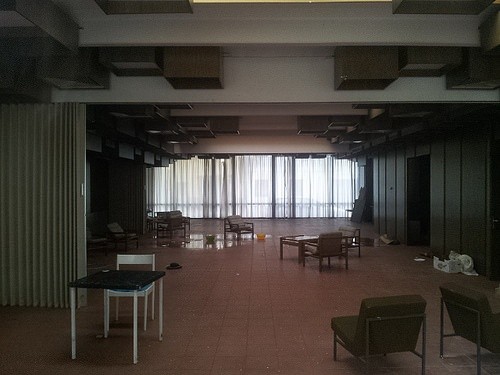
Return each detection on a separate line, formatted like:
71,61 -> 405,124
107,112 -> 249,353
146,210 -> 190,239
438,282 -> 500,375
224,215 -> 254,241
337,224 -> 361,259
331,294 -> 427,374
107,254 -> 155,331
303,231 -> 348,272
87,222 -> 139,258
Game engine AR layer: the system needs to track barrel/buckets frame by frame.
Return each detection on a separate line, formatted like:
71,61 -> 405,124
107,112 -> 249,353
257,233 -> 266,241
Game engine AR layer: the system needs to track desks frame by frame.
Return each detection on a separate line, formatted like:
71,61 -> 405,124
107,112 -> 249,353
67,269 -> 166,364
153,220 -> 189,238
280,234 -> 323,265
